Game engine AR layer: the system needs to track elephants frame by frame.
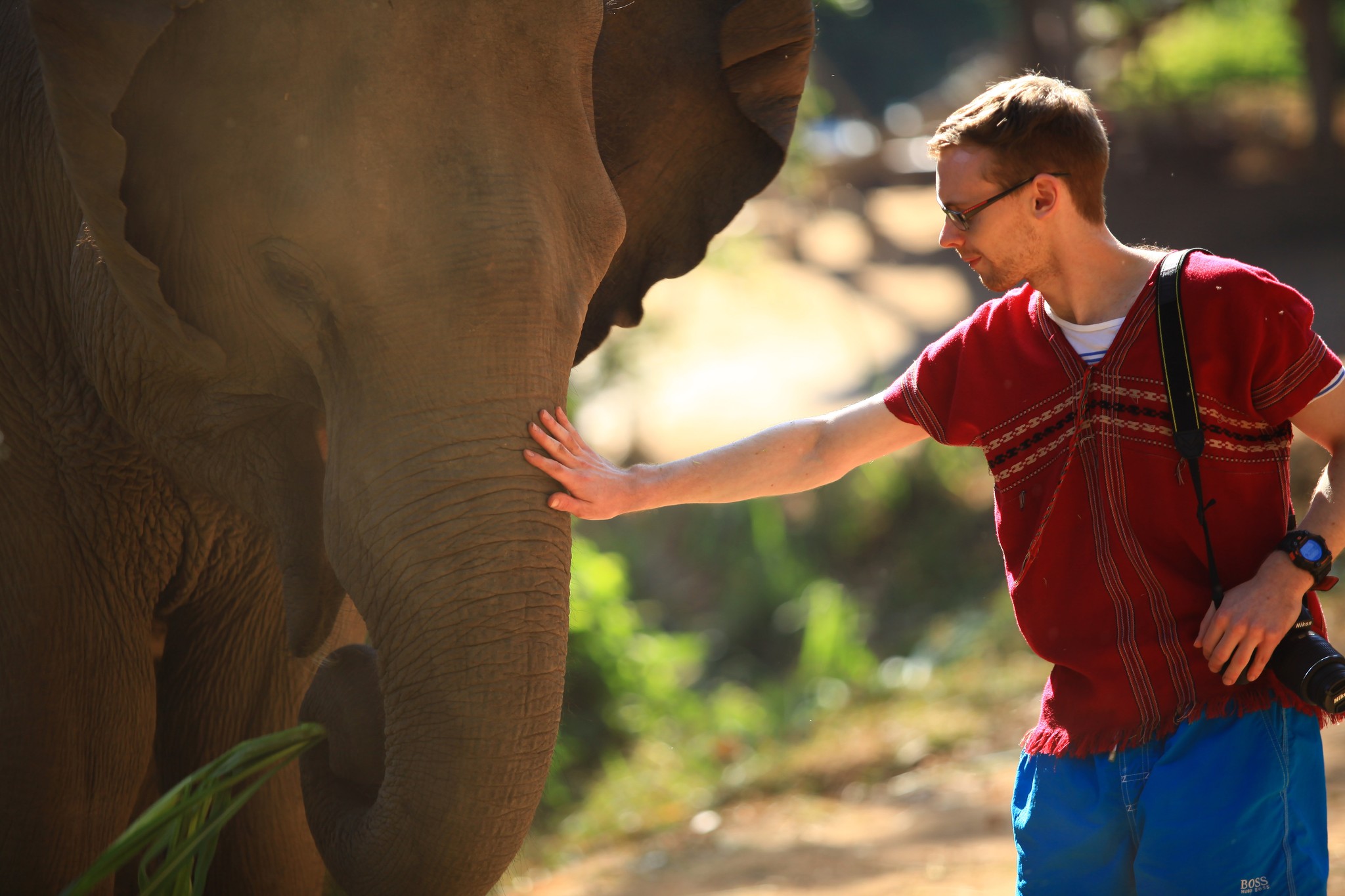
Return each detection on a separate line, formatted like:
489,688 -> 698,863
1,0 -> 818,896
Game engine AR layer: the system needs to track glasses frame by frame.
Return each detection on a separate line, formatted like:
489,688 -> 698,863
942,170 -> 1071,231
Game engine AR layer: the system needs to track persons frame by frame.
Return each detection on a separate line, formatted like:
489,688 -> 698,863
523,74 -> 1345,896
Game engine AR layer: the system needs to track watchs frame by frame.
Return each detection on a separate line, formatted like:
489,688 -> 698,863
1274,529 -> 1339,591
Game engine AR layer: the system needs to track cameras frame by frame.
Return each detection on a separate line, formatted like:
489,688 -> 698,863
1266,605 -> 1345,716
